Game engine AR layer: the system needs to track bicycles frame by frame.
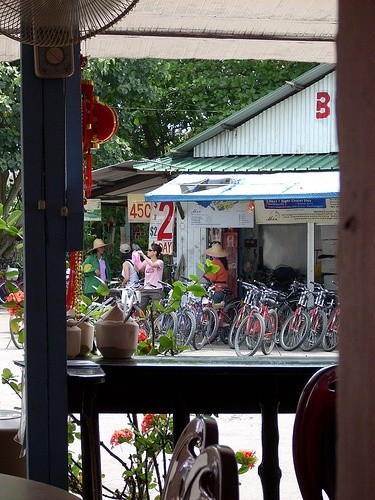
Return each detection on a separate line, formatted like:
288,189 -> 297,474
66,262 -> 230,353
229,262 -> 340,356
0,281 -> 23,304
0,309 -> 26,350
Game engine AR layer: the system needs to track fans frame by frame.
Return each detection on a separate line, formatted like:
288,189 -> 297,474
1,0 -> 140,79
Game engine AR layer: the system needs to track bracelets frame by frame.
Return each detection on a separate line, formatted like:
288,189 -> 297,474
144,256 -> 147,260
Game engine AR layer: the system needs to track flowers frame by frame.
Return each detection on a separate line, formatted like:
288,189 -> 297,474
0,261 -> 258,499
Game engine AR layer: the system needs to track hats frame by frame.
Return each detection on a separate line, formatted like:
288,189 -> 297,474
206,243 -> 229,258
120,244 -> 131,253
87,238 -> 108,253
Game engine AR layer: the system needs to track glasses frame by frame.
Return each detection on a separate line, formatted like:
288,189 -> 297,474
149,250 -> 156,252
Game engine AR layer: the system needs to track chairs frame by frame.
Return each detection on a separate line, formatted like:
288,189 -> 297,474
291,363 -> 337,500
0,418 -> 239,500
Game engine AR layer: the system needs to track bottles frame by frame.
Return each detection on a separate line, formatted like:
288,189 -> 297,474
77,314 -> 94,356
65,318 -> 82,359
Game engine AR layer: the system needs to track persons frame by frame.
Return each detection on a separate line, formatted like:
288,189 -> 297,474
82,238 -> 113,303
133,241 -> 165,323
202,242 -> 230,342
119,242 -> 138,308
